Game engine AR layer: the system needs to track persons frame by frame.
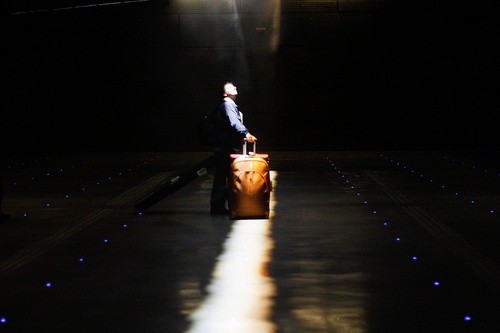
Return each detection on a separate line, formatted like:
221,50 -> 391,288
210,82 -> 257,213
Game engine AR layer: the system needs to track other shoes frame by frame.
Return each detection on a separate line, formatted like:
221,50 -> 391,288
210,207 -> 230,214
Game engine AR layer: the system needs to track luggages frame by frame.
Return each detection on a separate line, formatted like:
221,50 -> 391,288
226,137 -> 272,219
